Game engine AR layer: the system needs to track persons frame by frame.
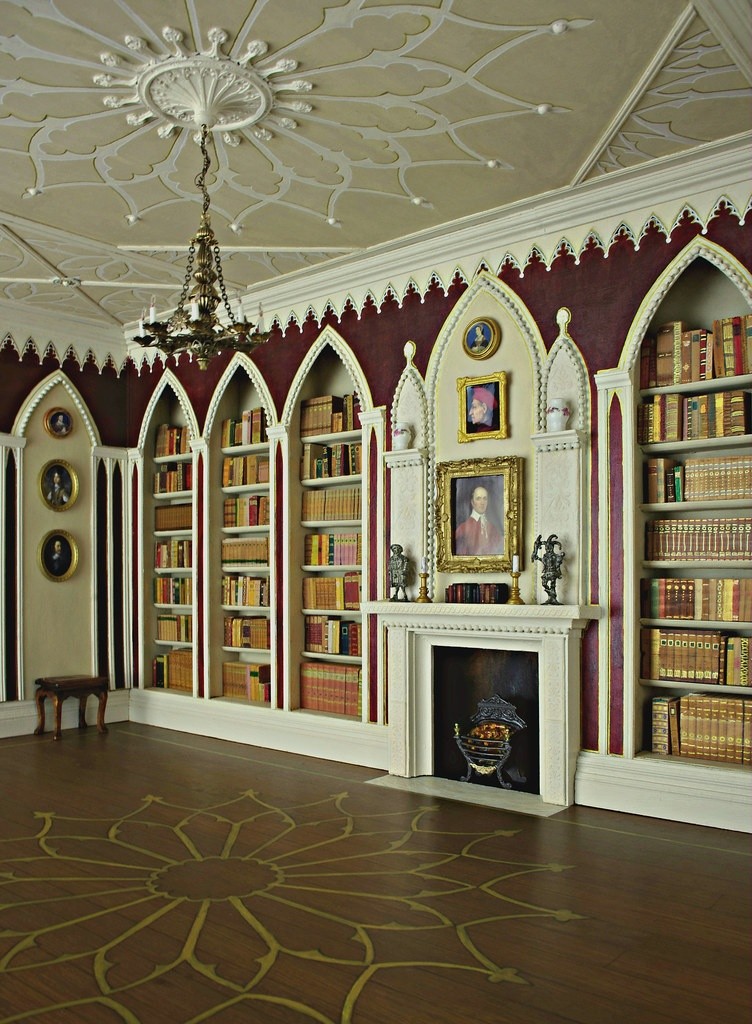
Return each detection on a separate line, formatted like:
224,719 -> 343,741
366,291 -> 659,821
46,470 -> 70,507
47,539 -> 67,576
52,415 -> 67,433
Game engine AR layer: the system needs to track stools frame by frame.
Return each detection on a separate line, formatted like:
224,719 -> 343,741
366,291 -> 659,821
33,675 -> 108,740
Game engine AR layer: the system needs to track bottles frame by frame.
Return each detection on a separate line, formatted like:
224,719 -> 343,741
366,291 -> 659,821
544,397 -> 570,433
392,422 -> 411,451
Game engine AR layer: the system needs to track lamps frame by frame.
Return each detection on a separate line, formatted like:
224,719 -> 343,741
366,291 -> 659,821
132,121 -> 269,372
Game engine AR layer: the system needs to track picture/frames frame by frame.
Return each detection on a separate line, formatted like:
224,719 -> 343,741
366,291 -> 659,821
462,316 -> 502,360
44,406 -> 73,439
36,459 -> 81,511
37,529 -> 78,582
435,456 -> 525,573
457,370 -> 509,442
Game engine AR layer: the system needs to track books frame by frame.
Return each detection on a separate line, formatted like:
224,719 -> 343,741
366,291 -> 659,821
445,583 -> 508,604
221,408 -> 271,704
300,395 -> 364,717
636,312 -> 752,766
153,423 -> 194,693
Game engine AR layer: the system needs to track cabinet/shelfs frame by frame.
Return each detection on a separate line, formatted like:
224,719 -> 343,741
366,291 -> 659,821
639,373 -> 752,773
216,443 -> 276,711
152,452 -> 200,696
299,428 -> 370,724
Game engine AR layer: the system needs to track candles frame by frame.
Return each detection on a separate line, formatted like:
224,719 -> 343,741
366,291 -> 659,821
512,555 -> 520,574
422,557 -> 427,574
